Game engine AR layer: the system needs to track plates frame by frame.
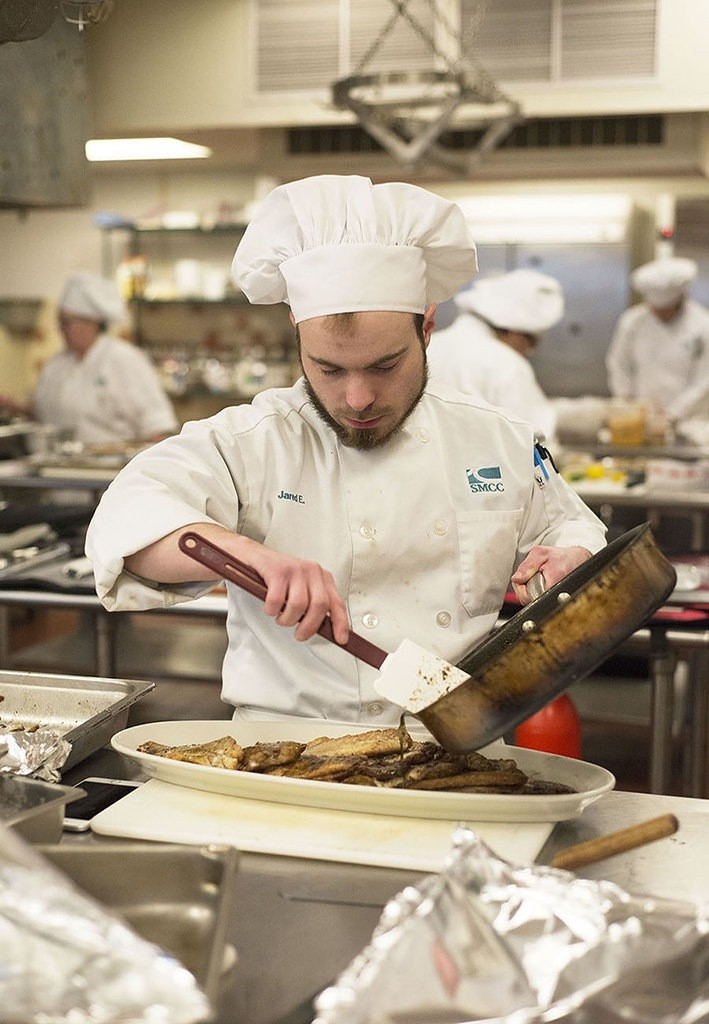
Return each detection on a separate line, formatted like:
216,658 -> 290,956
103,719 -> 616,823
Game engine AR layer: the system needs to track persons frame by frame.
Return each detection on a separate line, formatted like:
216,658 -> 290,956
606,256 -> 709,442
34,271 -> 180,453
85,175 -> 608,744
424,266 -> 565,455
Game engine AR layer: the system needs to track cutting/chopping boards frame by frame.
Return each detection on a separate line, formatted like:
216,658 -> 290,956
90,775 -> 559,878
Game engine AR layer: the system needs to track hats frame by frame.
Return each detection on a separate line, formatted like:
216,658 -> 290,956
232,173 -> 477,325
60,270 -> 128,321
627,257 -> 697,309
451,267 -> 566,336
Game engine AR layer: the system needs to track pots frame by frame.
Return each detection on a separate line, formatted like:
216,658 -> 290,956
412,521 -> 679,751
